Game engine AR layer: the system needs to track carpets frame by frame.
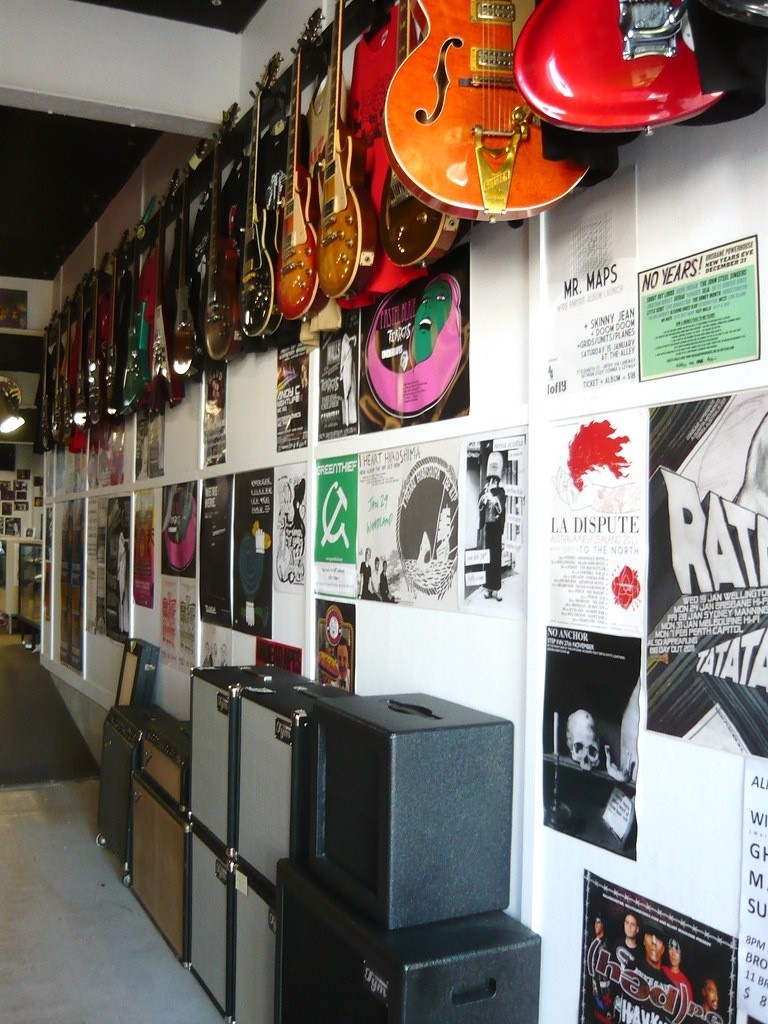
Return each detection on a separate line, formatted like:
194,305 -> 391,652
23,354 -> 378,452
0,644 -> 100,788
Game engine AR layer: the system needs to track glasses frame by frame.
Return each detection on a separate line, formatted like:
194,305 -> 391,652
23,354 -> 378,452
669,938 -> 680,953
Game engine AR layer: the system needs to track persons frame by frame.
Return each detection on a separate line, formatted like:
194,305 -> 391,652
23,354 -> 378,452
324,636 -> 350,692
475,450 -> 506,602
356,549 -> 398,603
584,912 -> 724,1024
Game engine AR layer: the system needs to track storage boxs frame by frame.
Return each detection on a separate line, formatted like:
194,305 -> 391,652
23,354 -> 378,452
187,664 -> 359,1024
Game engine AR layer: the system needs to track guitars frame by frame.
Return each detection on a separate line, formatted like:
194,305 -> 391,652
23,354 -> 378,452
30,0 -> 767,454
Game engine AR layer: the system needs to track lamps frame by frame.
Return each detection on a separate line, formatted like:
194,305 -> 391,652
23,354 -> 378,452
0,381 -> 25,433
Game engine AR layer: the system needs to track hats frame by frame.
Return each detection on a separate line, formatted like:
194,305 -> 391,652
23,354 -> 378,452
637,916 -> 668,953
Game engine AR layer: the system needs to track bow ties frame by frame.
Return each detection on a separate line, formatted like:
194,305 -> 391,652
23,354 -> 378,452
339,679 -> 347,687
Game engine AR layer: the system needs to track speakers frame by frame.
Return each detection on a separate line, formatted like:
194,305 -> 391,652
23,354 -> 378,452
96,664 -> 541,1024
